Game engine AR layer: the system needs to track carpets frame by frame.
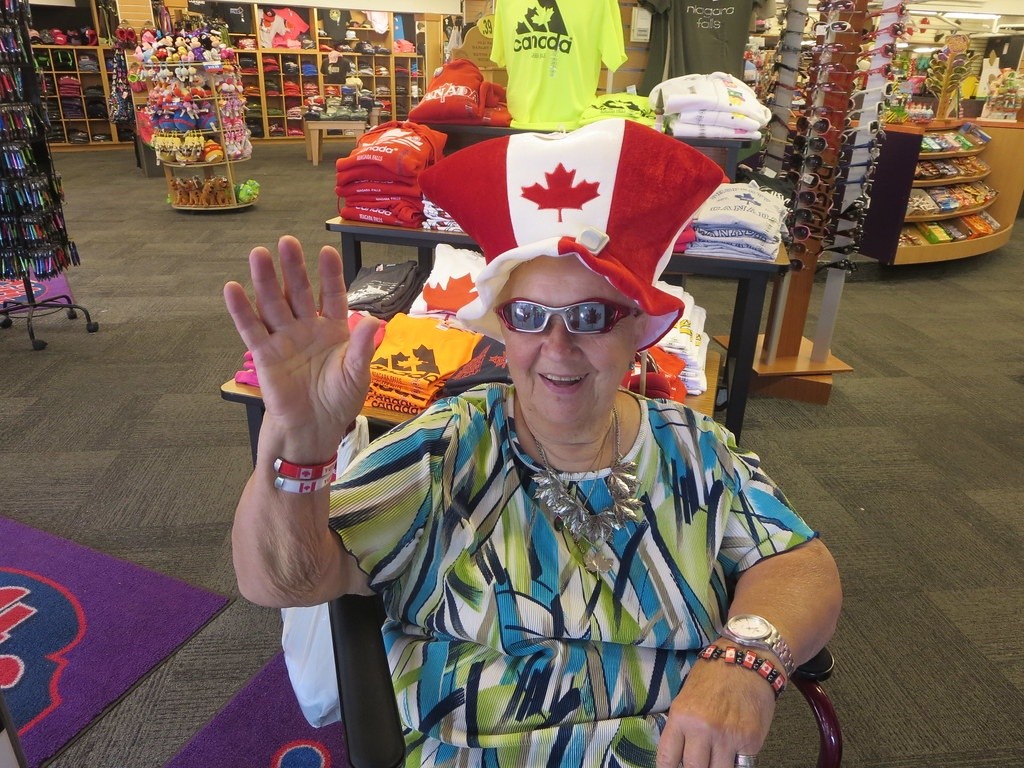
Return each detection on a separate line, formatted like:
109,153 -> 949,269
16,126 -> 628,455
0,514 -> 238,768
163,649 -> 354,768
0,265 -> 73,314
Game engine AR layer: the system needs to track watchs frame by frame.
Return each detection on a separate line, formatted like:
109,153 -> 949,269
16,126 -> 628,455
721,614 -> 796,678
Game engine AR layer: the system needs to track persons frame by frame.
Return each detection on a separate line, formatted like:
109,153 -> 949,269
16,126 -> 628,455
225,119 -> 844,768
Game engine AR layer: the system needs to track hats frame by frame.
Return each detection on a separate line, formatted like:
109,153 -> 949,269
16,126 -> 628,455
29,26 -> 98,46
417,118 -> 725,352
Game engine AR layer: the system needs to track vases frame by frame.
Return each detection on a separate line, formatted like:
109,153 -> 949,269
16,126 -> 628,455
962,98 -> 986,117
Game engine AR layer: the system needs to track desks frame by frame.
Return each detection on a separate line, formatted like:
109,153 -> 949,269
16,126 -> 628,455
300,105 -> 382,160
419,122 -> 750,184
325,215 -> 791,448
305,120 -> 366,166
220,350 -> 721,474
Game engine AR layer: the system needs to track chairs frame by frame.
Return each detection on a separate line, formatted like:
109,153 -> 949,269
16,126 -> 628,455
327,594 -> 844,767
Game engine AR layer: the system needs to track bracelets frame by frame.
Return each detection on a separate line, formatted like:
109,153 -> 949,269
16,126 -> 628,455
273,454 -> 339,494
696,645 -> 785,700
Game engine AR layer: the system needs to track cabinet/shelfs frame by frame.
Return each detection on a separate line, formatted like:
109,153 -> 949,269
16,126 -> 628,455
857,117 -> 1023,279
187,0 -> 427,145
29,0 -> 136,152
144,63 -> 258,210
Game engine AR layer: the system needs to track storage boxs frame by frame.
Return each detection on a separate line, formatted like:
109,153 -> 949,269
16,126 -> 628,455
907,180 -> 997,216
921,122 -> 991,153
915,214 -> 994,244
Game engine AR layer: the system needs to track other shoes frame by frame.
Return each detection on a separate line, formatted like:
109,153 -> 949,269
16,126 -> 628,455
138,71 -> 148,90
116,26 -> 128,50
125,27 -> 137,50
127,72 -> 141,93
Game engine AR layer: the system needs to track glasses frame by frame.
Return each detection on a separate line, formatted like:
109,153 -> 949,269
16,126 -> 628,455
733,1 -> 906,274
493,297 -> 642,335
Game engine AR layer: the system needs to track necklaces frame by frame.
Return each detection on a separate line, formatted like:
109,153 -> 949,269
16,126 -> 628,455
530,405 -> 645,581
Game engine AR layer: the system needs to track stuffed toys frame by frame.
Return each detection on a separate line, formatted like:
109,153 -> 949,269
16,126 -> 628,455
130,27 -> 261,208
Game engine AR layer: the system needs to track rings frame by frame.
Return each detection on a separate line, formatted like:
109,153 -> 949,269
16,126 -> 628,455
735,754 -> 759,768
